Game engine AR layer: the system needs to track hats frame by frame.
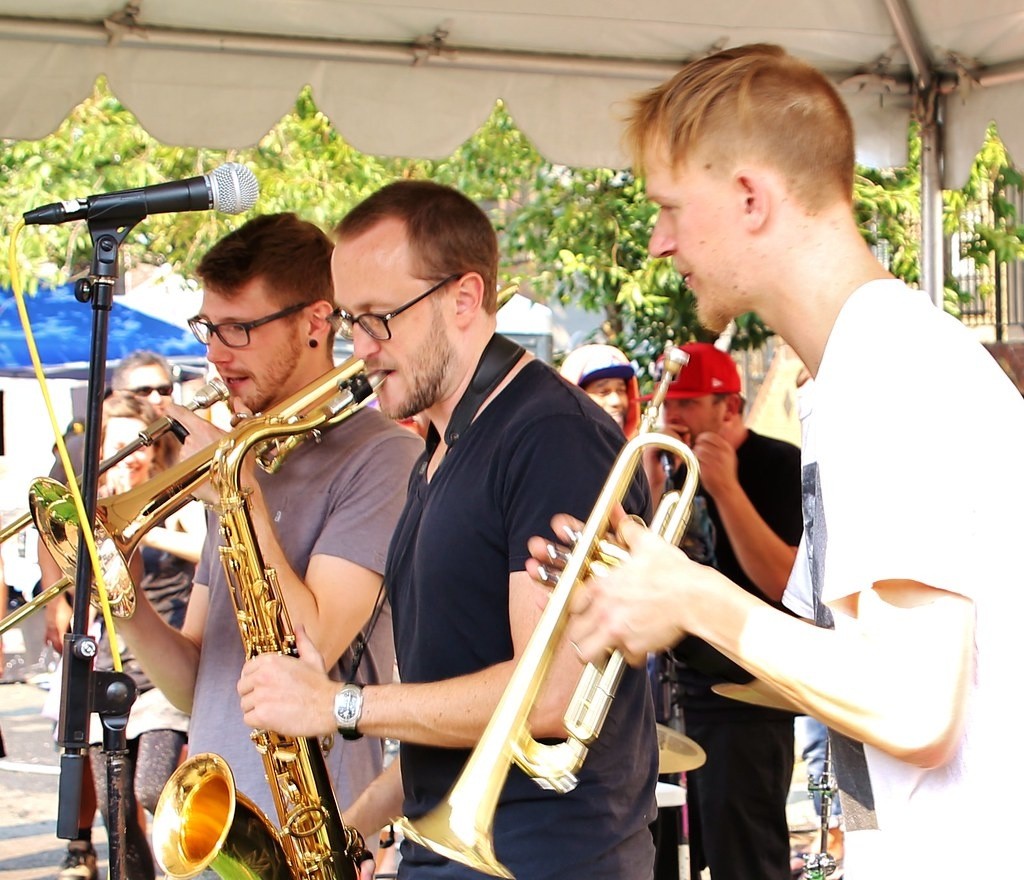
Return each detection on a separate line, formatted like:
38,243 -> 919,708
629,343 -> 742,403
577,356 -> 635,387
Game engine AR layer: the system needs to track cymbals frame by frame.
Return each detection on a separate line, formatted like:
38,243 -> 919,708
710,679 -> 805,713
657,722 -> 706,775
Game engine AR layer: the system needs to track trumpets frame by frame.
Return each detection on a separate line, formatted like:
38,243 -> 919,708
393,348 -> 700,879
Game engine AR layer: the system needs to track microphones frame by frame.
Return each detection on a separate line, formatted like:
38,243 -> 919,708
20,163 -> 259,217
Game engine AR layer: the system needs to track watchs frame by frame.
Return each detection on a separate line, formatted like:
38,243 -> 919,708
331,680 -> 366,741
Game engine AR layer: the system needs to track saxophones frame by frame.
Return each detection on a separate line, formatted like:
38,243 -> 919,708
152,369 -> 390,879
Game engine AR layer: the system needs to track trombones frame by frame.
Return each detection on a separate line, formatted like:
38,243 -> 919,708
0,283 -> 521,632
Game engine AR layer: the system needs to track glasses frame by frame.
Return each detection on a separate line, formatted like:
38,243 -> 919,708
325,272 -> 462,341
118,384 -> 173,397
187,303 -> 308,349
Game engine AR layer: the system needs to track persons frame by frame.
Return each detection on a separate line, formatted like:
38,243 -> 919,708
100,211 -> 430,880
522,41 -> 1024,880
0,350 -> 211,880
233,180 -> 663,880
